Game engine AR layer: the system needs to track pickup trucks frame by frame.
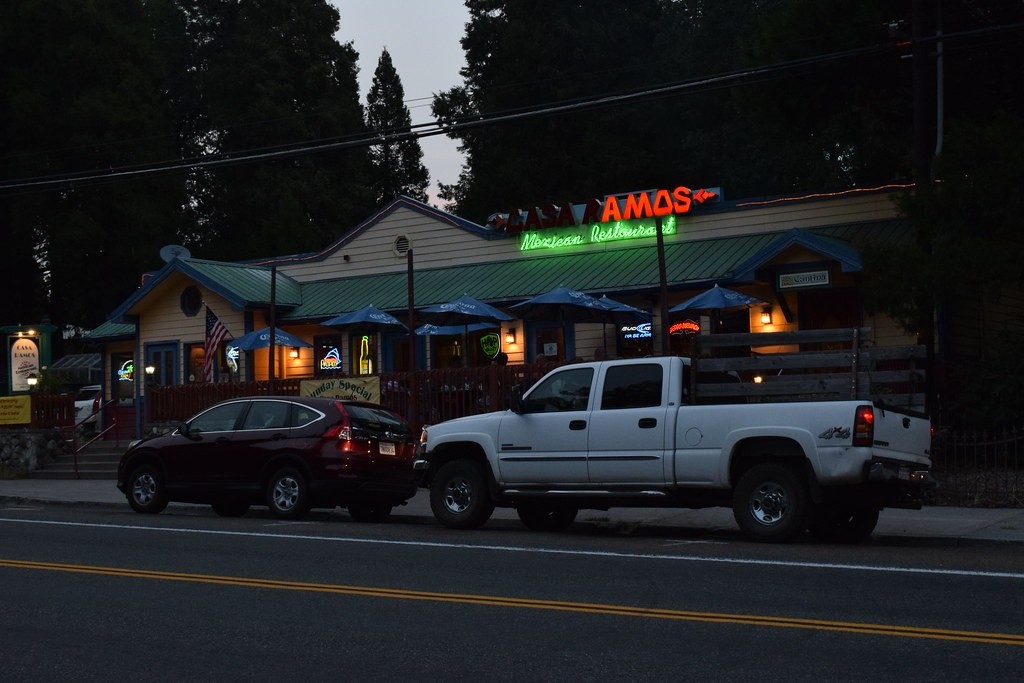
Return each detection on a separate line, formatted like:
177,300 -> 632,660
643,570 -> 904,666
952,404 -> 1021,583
413,355 -> 932,540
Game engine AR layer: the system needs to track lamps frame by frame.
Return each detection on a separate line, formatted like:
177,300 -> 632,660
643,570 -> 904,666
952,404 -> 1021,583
25,373 -> 39,391
760,304 -> 771,324
145,363 -> 156,385
506,328 -> 517,344
289,345 -> 300,358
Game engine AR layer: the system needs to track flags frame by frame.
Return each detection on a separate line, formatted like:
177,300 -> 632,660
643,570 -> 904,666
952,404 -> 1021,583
204,306 -> 228,385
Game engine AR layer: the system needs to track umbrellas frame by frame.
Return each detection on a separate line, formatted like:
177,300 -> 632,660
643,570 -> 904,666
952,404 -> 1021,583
417,293 -> 517,323
668,284 -> 773,312
319,304 -> 411,336
230,326 -> 315,351
576,293 -> 659,324
508,283 -> 619,312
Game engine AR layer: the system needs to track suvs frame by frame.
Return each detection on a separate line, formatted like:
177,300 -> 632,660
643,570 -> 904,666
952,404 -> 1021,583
116,393 -> 418,524
72,386 -> 104,431
